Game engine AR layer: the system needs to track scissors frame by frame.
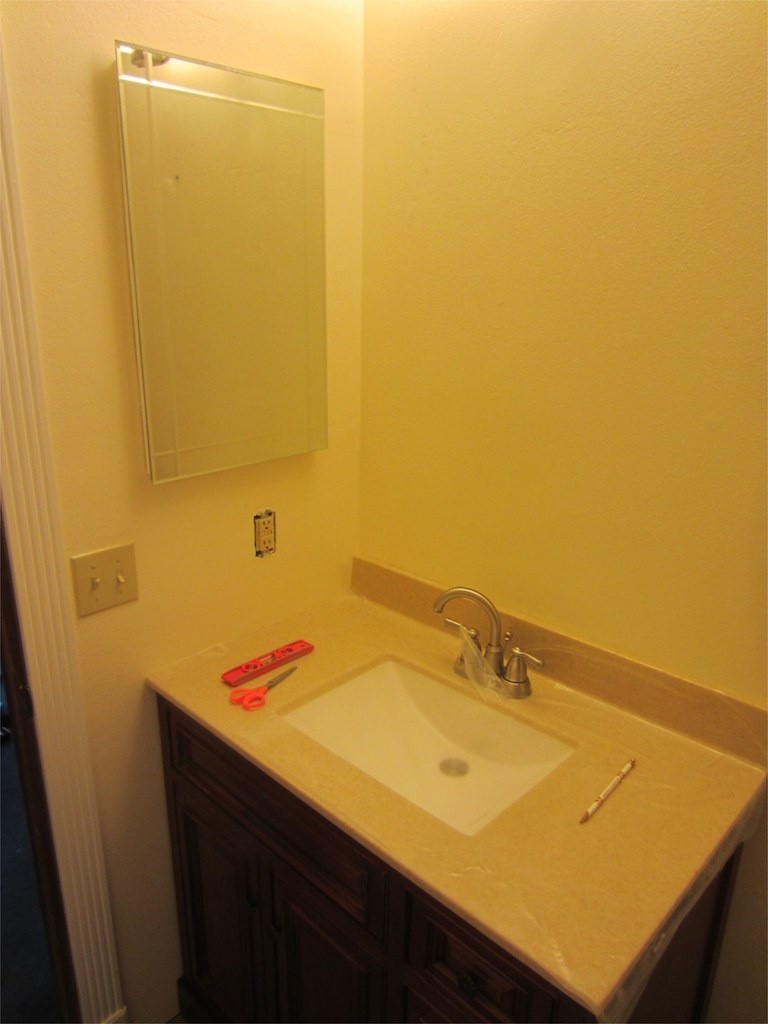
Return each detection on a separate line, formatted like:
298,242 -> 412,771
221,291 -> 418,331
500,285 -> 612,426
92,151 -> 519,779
229,666 -> 297,708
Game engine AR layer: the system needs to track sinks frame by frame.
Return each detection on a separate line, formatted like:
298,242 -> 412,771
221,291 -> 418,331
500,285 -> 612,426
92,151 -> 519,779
274,653 -> 579,839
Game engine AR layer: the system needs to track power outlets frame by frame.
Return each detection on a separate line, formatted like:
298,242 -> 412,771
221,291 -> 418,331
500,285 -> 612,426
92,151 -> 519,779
256,516 -> 275,553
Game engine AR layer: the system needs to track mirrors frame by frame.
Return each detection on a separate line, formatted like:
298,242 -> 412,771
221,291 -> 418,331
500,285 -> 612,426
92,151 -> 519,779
116,41 -> 329,488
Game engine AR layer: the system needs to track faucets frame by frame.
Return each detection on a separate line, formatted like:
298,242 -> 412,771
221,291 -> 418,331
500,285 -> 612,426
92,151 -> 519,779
433,587 -> 512,690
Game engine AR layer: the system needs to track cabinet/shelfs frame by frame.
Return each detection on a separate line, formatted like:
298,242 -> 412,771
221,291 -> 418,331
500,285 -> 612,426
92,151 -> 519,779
157,690 -> 595,1024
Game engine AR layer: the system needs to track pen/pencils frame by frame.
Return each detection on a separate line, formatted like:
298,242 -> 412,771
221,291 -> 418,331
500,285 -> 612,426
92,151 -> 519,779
579,757 -> 636,824
